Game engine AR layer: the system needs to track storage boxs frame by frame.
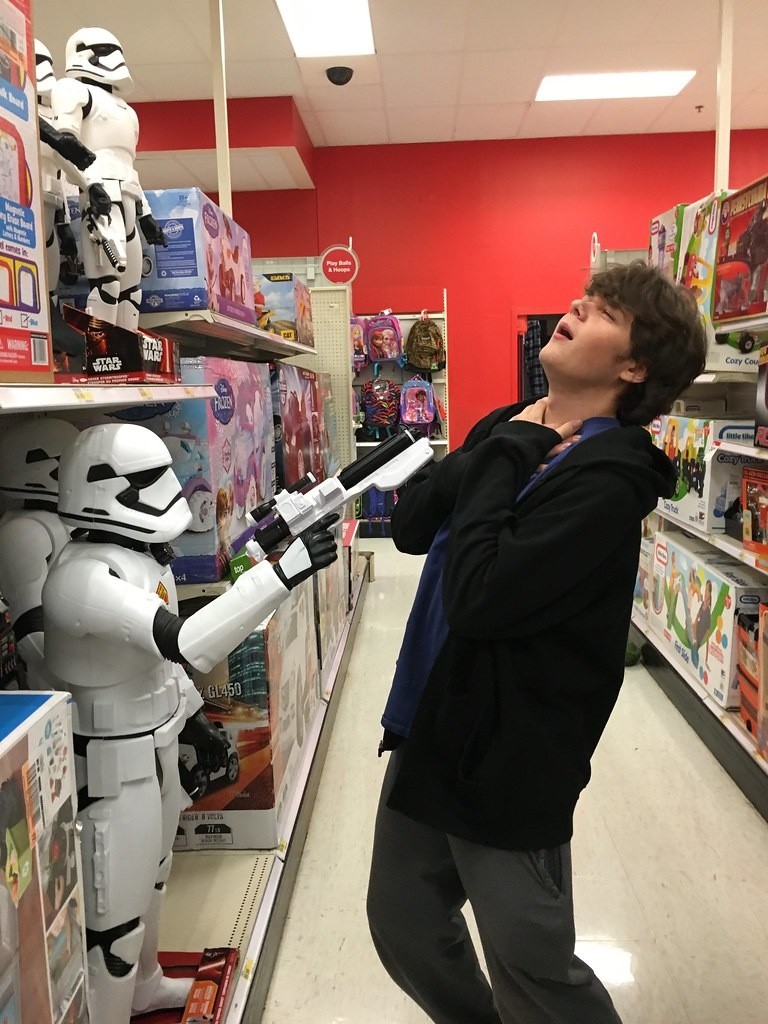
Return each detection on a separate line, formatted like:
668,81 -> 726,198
631,179 -> 768,760
0,0 -> 348,1024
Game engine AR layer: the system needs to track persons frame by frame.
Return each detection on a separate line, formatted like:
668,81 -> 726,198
39,423 -> 338,1024
50,27 -> 169,330
0,412 -> 80,694
33,37 -> 85,312
363,257 -> 708,1024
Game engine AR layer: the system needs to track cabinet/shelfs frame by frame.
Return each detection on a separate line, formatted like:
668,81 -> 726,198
590,167 -> 768,825
0,0 -> 372,1024
351,288 -> 449,538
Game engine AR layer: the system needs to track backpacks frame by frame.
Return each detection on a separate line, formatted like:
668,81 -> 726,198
354,476 -> 400,536
351,373 -> 400,439
404,309 -> 445,383
366,310 -> 407,376
399,375 -> 438,440
349,311 -> 369,377
